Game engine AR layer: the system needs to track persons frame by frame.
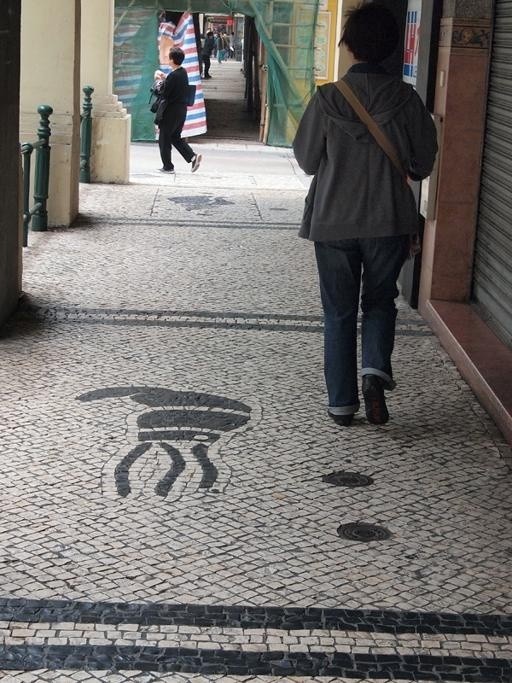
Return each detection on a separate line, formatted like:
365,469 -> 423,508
292,3 -> 441,427
151,45 -> 203,173
213,30 -> 234,62
200,33 -> 206,68
202,31 -> 215,78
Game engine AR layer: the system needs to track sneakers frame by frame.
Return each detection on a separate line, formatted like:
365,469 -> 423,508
327,410 -> 353,426
155,166 -> 175,174
191,155 -> 201,172
362,374 -> 388,425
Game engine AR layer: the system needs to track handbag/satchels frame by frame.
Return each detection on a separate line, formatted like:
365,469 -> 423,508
185,85 -> 196,105
